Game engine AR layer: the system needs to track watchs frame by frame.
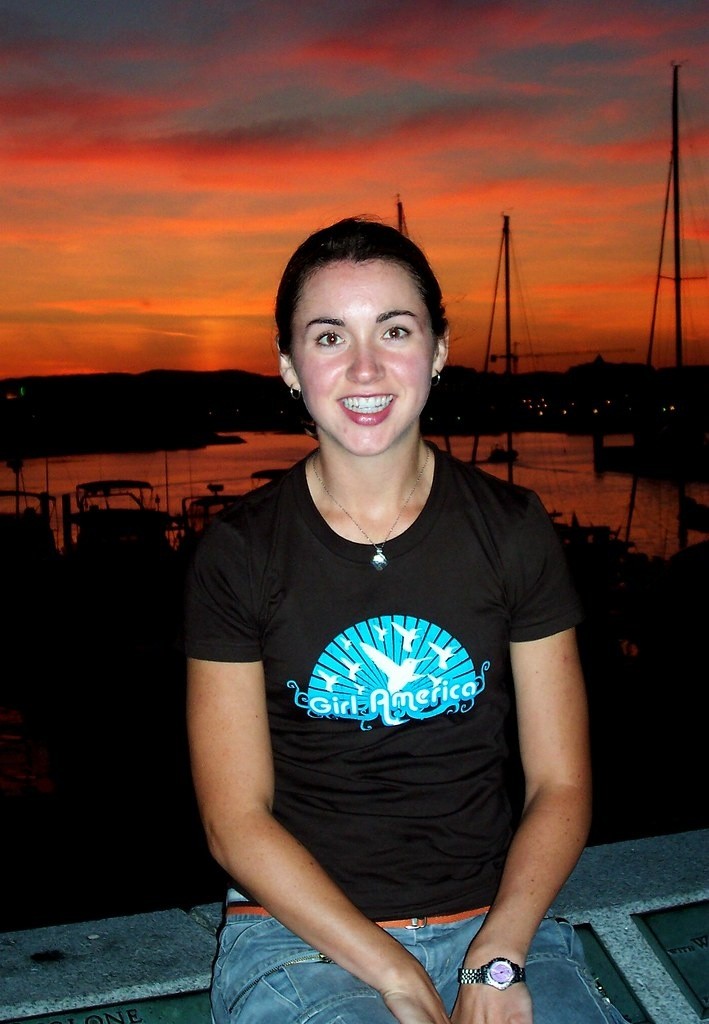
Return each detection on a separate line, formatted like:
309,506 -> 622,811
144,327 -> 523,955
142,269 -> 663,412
456,957 -> 526,991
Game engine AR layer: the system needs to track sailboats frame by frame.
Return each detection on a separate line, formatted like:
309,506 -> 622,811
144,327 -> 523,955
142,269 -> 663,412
395,61 -> 709,546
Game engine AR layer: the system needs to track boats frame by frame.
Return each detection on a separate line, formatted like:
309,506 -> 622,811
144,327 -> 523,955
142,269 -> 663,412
59,447 -> 174,557
0,451 -> 60,560
175,451 -> 250,560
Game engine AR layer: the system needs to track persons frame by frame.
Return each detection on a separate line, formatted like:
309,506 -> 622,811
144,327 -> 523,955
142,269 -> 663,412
174,216 -> 635,1024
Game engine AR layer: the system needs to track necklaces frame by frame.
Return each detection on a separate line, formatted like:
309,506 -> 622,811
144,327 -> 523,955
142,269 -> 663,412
313,450 -> 431,572
